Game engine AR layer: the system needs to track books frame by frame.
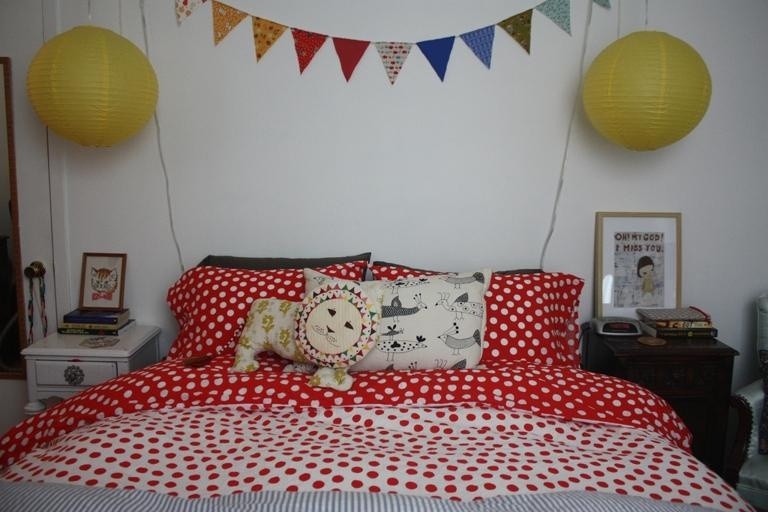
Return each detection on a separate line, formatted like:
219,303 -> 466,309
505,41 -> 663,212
56,310 -> 137,336
636,306 -> 718,339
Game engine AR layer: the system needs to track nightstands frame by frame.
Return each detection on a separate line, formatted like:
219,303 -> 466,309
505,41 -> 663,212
579,320 -> 739,483
20,323 -> 159,416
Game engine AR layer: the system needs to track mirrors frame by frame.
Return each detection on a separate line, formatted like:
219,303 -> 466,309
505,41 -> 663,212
0,55 -> 28,381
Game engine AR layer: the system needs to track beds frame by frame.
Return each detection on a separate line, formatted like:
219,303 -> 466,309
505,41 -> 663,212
1,253 -> 760,512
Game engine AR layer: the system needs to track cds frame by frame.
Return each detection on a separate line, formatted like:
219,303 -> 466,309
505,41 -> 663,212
637,336 -> 666,346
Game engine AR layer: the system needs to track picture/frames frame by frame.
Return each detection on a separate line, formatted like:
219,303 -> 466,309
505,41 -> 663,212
594,210 -> 683,320
79,252 -> 127,313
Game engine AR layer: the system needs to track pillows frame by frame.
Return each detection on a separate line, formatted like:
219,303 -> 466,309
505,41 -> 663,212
166,260 -> 367,365
302,265 -> 493,373
197,253 -> 371,281
368,262 -> 585,370
234,281 -> 384,391
